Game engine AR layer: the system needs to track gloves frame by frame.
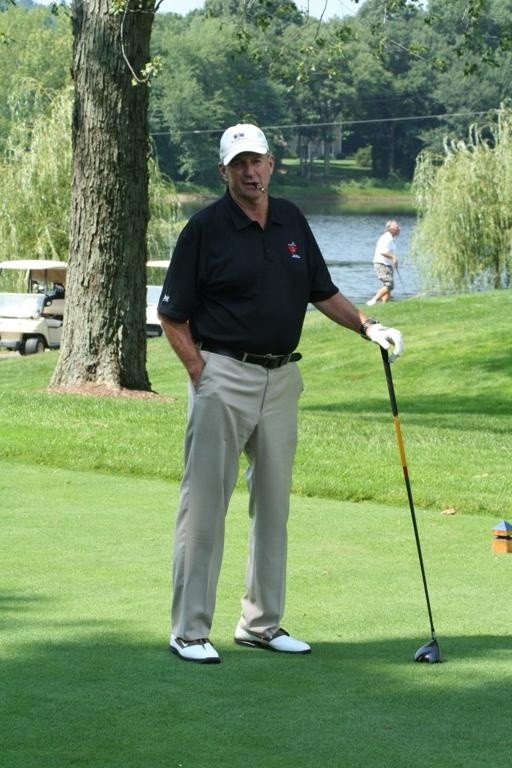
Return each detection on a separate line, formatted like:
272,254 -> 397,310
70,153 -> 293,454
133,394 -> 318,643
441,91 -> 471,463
365,322 -> 405,364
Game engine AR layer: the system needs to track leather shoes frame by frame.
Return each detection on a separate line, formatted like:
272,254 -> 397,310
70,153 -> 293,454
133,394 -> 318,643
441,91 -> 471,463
233,622 -> 315,654
167,628 -> 221,665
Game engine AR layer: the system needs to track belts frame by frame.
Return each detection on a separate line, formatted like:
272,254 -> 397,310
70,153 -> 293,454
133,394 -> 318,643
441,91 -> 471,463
198,342 -> 304,370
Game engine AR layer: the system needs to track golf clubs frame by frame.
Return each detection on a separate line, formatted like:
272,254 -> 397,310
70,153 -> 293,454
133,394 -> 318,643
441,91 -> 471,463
381,349 -> 444,664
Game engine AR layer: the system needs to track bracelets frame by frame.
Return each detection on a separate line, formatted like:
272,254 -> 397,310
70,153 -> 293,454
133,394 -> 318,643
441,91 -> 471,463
360,318 -> 380,342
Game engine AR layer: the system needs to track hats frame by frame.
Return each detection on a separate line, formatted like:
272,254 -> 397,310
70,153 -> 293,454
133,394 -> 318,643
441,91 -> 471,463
219,123 -> 271,168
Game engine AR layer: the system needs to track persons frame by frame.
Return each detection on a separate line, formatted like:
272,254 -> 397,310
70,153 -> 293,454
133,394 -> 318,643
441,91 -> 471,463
156,122 -> 403,665
365,219 -> 401,308
